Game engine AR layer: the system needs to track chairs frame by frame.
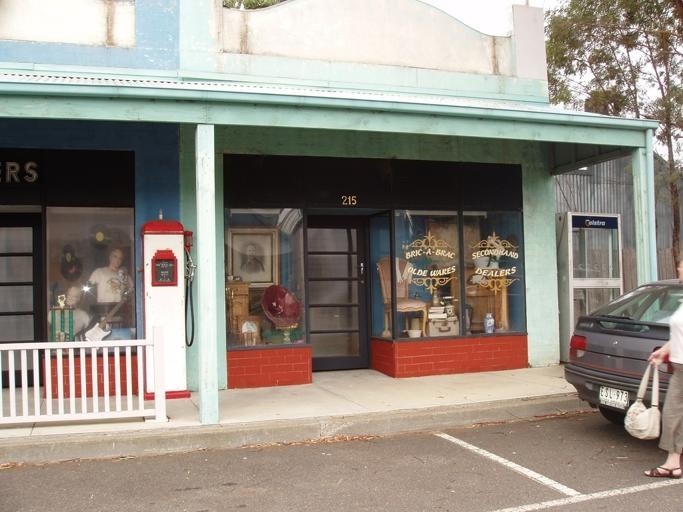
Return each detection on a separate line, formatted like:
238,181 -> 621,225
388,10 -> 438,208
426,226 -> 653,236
376,257 -> 429,338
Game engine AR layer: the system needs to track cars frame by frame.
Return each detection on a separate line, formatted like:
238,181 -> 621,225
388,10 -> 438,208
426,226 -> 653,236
564,278 -> 682,443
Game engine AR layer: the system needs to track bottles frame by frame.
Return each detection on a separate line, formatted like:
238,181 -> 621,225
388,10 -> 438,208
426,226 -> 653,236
442,296 -> 455,316
483,313 -> 494,333
464,304 -> 473,337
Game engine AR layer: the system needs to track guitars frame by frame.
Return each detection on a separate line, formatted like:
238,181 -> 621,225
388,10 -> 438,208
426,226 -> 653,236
75,296 -> 126,354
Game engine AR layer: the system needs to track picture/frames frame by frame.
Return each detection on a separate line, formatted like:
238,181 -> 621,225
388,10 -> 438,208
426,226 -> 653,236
227,226 -> 280,290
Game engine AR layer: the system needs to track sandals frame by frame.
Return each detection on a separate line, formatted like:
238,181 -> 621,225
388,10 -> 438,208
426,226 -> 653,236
645,466 -> 681,478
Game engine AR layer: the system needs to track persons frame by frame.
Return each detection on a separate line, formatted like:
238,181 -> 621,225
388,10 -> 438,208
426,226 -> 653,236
240,241 -> 264,275
87,249 -> 132,325
640,252 -> 683,479
59,243 -> 81,282
48,286 -> 90,337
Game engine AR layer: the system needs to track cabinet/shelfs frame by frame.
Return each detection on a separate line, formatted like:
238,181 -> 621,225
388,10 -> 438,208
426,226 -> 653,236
451,267 -> 508,333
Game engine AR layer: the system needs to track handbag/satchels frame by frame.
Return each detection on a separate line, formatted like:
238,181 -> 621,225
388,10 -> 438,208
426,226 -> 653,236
624,400 -> 660,440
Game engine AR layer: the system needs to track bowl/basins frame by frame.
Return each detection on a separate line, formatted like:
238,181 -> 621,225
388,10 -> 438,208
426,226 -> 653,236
406,330 -> 421,338
